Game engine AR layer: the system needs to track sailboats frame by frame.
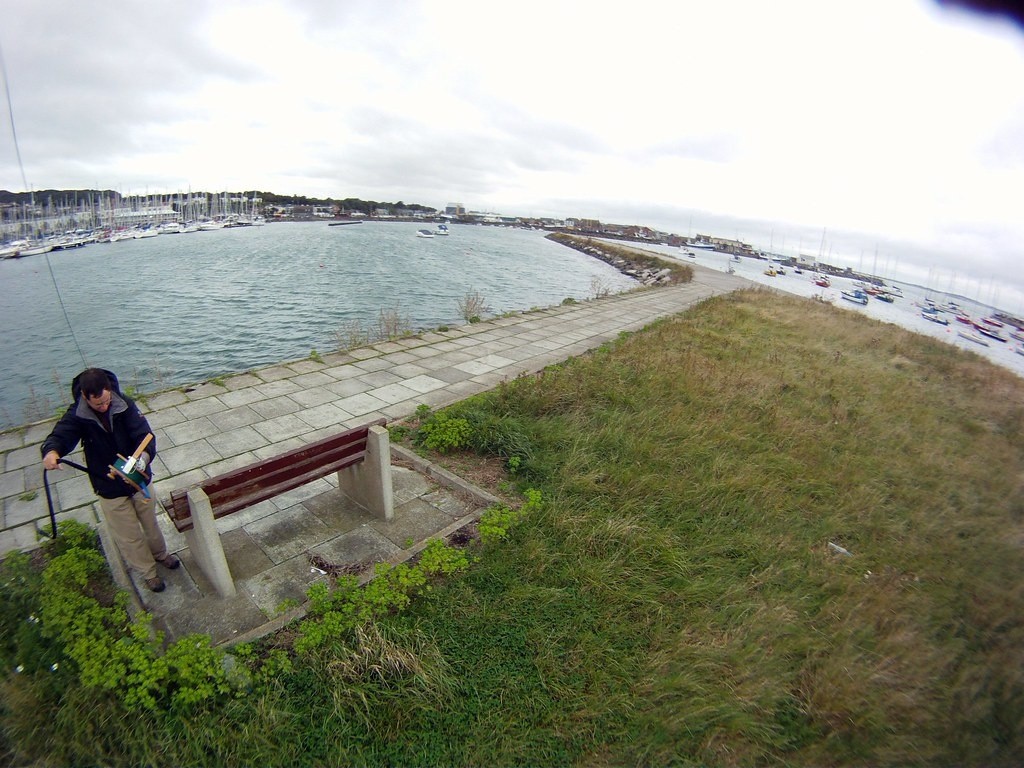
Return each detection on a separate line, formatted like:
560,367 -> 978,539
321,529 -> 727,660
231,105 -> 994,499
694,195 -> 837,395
763,229 -> 777,277
794,239 -> 804,274
0,183 -> 266,257
854,243 -> 904,304
812,227 -> 831,288
775,237 -> 787,276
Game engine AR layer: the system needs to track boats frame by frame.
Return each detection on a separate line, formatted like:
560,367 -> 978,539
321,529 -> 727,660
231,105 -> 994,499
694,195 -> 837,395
731,255 -> 743,263
957,329 -> 989,347
433,224 -> 450,235
416,229 -> 435,238
688,252 -> 696,258
914,267 -> 1024,355
685,238 -> 716,251
679,246 -> 691,255
839,286 -> 869,305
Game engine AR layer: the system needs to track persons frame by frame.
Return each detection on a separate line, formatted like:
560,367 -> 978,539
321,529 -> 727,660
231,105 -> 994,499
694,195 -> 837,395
40,368 -> 179,592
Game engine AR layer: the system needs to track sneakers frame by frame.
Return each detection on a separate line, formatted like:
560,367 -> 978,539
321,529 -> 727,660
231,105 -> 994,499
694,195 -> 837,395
145,576 -> 164,593
157,554 -> 179,569
